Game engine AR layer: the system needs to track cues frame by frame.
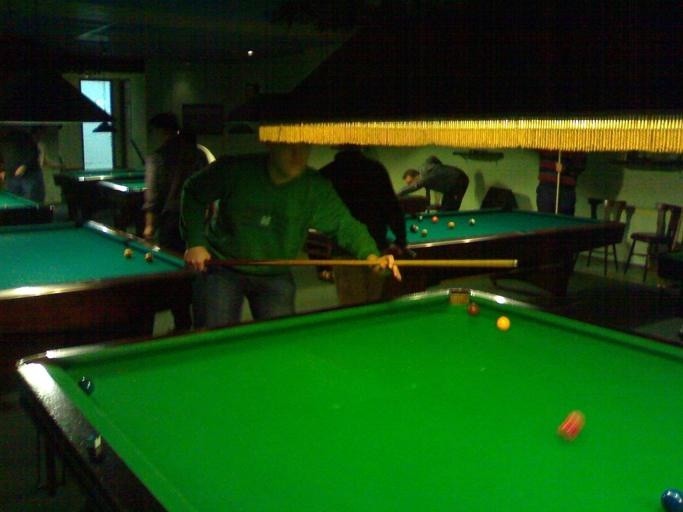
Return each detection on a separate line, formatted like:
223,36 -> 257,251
205,258 -> 518,268
555,149 -> 560,215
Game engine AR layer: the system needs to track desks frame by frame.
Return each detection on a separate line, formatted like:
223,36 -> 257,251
305,208 -> 627,307
10,287 -> 683,512
0,218 -> 197,413
301,194 -> 432,284
0,162 -> 144,239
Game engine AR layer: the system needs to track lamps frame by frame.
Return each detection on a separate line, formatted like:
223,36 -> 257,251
91,119 -> 116,132
259,0 -> 680,151
1,0 -> 112,123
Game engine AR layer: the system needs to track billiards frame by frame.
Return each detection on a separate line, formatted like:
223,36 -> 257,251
469,218 -> 476,224
497,317 -> 509,330
145,253 -> 153,262
410,225 -> 418,231
661,490 -> 682,510
78,378 -> 94,395
420,229 -> 427,237
419,216 -> 423,221
560,412 -> 581,435
123,248 -> 131,257
432,216 -> 438,222
468,303 -> 479,316
448,222 -> 455,228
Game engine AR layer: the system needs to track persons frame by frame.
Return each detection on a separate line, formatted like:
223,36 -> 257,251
26,130 -> 43,174
305,142 -> 405,303
396,153 -> 470,210
534,147 -> 589,217
177,127 -> 402,340
141,110 -> 219,335
0,127 -> 46,204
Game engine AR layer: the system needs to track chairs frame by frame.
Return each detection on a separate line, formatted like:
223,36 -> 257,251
476,186 -> 517,213
622,205 -> 680,286
584,196 -> 626,275
